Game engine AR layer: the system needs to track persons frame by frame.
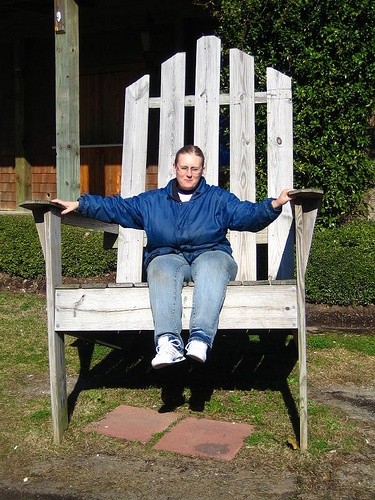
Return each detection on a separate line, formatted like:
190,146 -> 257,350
50,146 -> 297,370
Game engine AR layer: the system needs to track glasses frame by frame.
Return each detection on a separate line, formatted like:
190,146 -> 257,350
176,165 -> 202,174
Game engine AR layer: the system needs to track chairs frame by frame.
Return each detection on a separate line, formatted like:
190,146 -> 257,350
18,35 -> 325,453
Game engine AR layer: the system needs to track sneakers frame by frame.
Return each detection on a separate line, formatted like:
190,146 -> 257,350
151,336 -> 186,369
185,340 -> 208,363
